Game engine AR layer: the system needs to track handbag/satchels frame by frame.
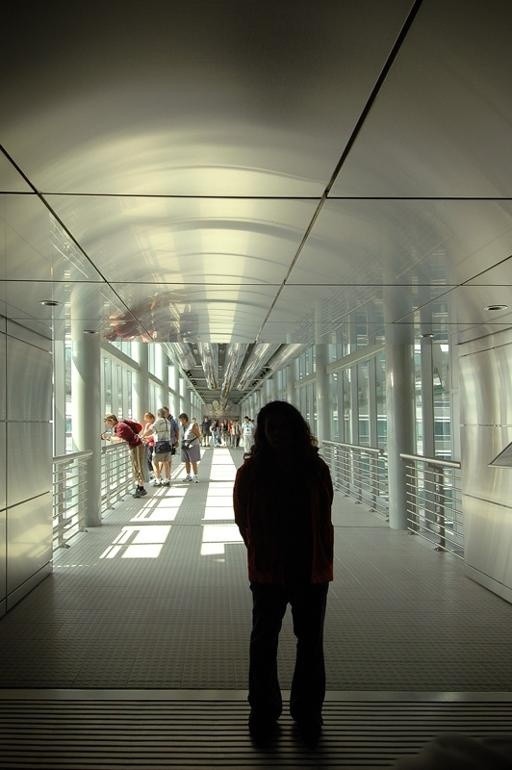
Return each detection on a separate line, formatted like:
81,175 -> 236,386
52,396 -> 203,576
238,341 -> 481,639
154,441 -> 171,452
171,444 -> 175,455
182,440 -> 192,450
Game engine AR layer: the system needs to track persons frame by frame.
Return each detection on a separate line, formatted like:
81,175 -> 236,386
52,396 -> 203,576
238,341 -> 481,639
234,398 -> 335,731
100,406 -> 256,499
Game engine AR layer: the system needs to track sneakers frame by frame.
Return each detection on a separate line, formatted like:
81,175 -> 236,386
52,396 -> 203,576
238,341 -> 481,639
183,475 -> 199,484
133,489 -> 146,498
153,481 -> 171,487
